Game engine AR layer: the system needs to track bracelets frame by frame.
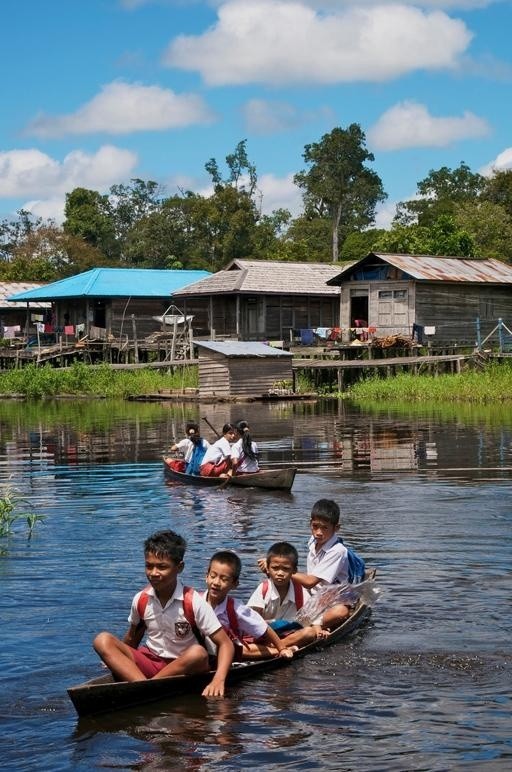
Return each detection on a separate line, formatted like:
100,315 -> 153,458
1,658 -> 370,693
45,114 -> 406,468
279,646 -> 287,652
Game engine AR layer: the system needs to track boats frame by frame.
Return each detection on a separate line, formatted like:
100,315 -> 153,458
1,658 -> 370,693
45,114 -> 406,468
162,455 -> 298,491
66,568 -> 377,717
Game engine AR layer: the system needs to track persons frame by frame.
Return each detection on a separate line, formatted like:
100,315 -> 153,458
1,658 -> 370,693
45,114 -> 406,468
198,551 -> 296,670
164,420 -> 213,473
199,423 -> 239,479
239,660 -> 311,752
162,475 -> 255,550
238,540 -> 333,661
91,527 -> 237,698
354,317 -> 368,341
226,418 -> 262,476
120,692 -> 244,771
257,499 -> 350,632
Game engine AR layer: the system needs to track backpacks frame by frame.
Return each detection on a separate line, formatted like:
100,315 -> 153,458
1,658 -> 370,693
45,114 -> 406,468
340,548 -> 366,610
189,437 -> 206,476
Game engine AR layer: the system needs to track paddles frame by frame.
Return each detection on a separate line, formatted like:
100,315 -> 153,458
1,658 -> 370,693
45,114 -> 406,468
216,477 -> 230,489
